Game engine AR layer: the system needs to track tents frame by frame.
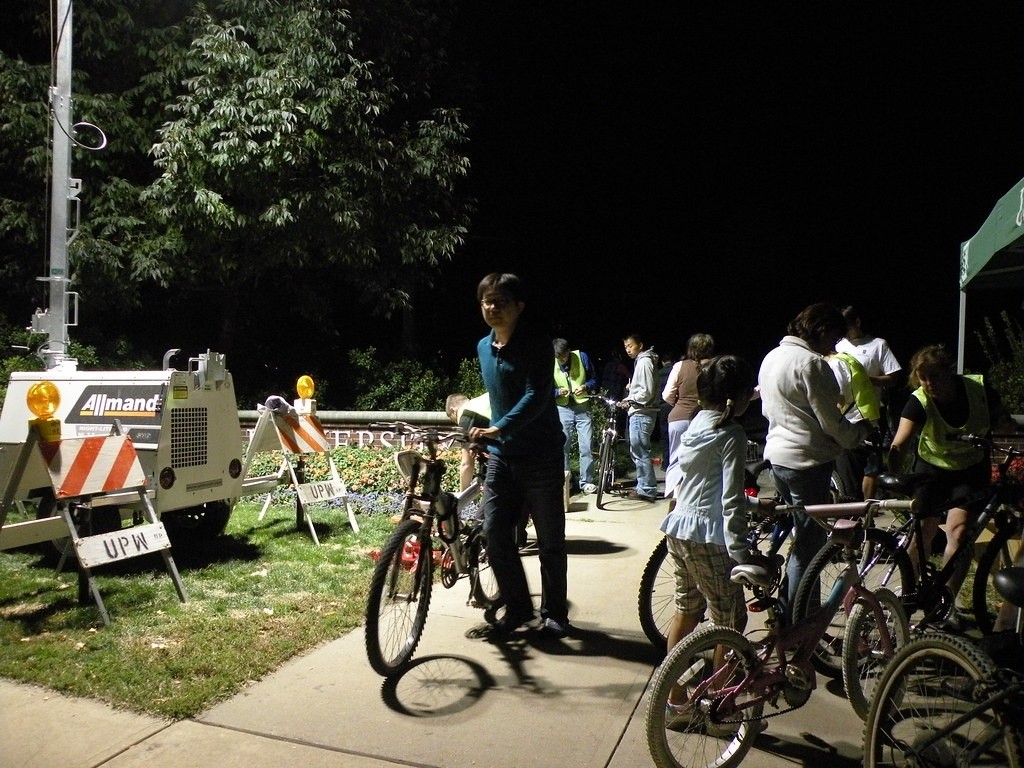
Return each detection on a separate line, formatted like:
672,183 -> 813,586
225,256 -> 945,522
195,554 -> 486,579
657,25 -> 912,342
956,176 -> 1024,375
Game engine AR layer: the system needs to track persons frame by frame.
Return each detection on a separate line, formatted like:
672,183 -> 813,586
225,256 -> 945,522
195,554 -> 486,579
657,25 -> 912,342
618,333 -> 661,503
663,355 -> 769,737
887,344 -> 1024,599
445,392 -> 529,547
941,526 -> 1024,705
750,302 -> 902,624
662,333 -> 715,515
552,338 -> 599,494
468,273 -> 569,646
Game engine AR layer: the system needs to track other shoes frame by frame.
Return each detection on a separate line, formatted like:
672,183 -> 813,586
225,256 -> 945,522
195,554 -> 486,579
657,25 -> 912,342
621,489 -> 658,502
938,612 -> 978,635
492,600 -> 536,635
942,667 -> 1024,709
705,710 -> 769,737
541,606 -> 569,639
785,632 -> 862,661
878,585 -> 918,610
581,482 -> 598,495
664,700 -> 705,729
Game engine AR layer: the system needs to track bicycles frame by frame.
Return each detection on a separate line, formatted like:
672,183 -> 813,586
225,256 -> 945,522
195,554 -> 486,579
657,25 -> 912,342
365,421 -> 520,677
638,427 -> 1024,768
583,394 -> 627,508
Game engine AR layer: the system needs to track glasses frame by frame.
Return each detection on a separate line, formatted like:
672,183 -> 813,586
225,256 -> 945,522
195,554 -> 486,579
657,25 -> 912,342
480,295 -> 514,308
